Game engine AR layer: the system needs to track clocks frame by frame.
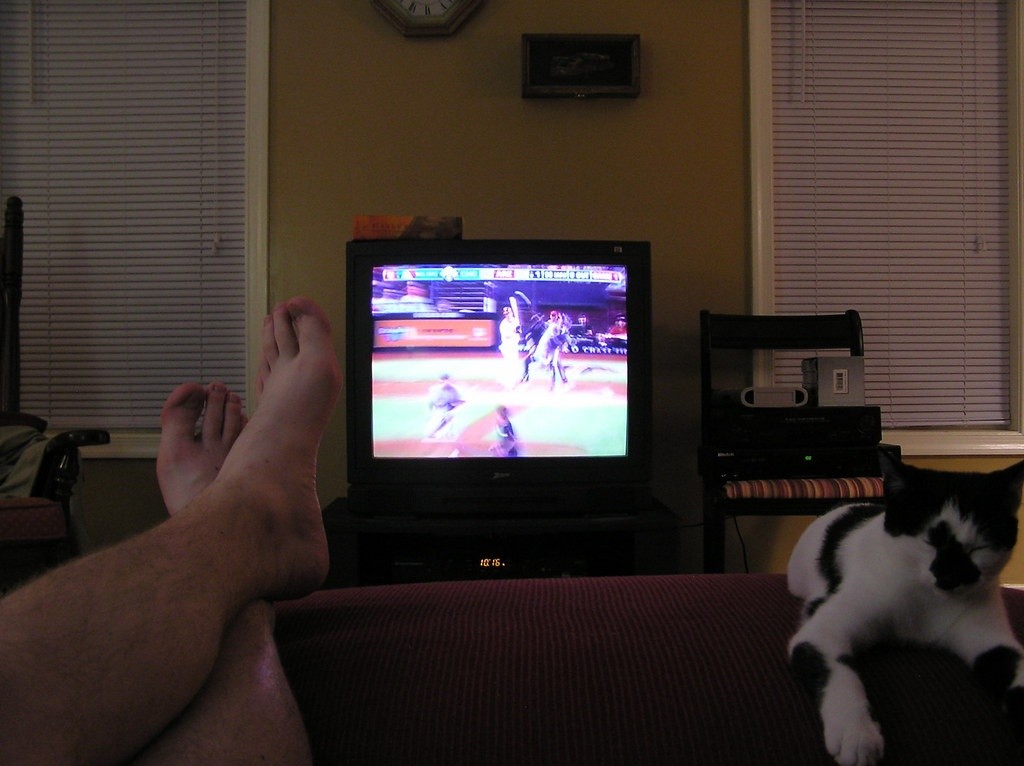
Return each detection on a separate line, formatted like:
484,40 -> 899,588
370,0 -> 481,38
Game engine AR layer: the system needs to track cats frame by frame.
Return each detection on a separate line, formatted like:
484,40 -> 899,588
786,445 -> 1023,765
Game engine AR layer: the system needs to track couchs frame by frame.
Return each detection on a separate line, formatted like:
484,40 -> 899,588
268,573 -> 1024,766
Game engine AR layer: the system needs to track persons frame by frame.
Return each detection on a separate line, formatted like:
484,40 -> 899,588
0,297 -> 341,766
373,265 -> 628,458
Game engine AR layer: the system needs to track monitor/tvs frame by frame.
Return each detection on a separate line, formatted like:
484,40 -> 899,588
345,239 -> 655,518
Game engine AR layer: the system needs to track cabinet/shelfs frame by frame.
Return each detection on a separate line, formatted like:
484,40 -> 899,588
323,496 -> 675,588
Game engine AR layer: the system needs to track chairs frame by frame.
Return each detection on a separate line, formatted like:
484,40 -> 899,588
701,308 -> 903,572
0,197 -> 111,593
